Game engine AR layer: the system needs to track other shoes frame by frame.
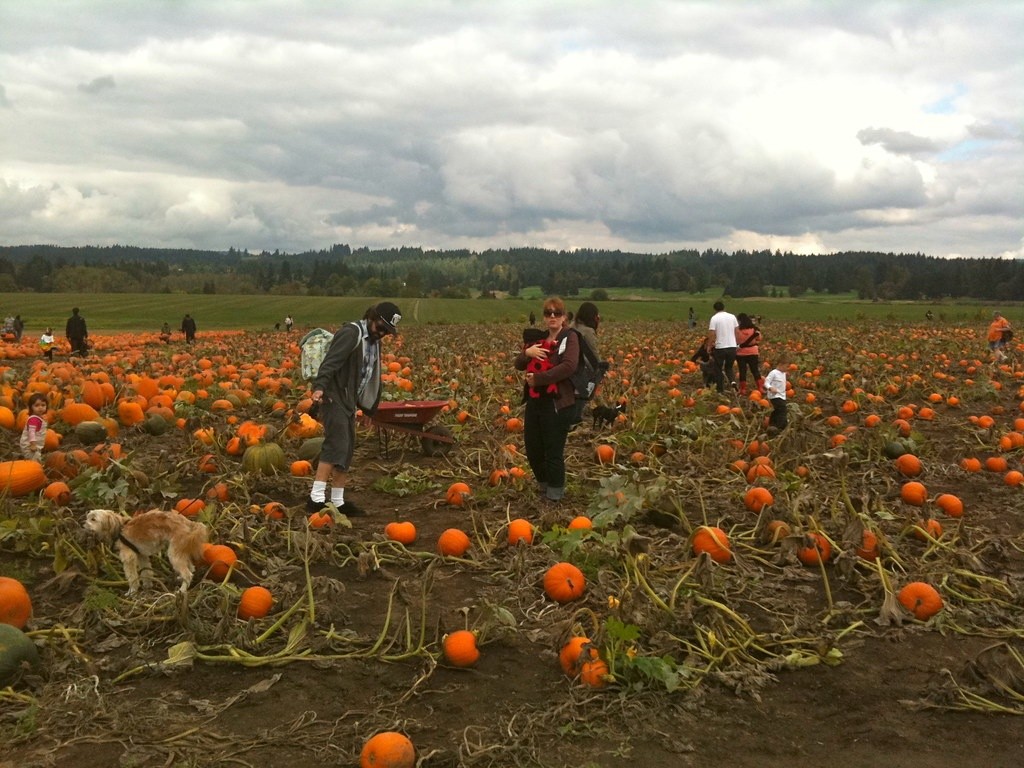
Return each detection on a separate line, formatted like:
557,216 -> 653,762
534,481 -> 548,498
732,384 -> 739,393
305,496 -> 329,516
543,485 -> 565,509
328,500 -> 367,517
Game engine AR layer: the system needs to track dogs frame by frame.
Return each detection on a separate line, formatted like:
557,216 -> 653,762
520,328 -> 561,404
592,399 -> 629,431
83,508 -> 206,596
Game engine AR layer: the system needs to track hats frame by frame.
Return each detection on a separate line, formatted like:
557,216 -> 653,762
375,302 -> 402,338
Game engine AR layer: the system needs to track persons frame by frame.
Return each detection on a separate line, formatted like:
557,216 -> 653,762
514,297 -> 579,499
735,313 -> 766,395
161,322 -> 170,344
691,302 -> 738,393
66,307 -> 88,351
567,302 -> 600,434
285,315 -> 293,331
926,310 -> 932,320
988,312 -> 1011,363
529,312 -> 535,325
41,327 -> 53,362
5,313 -> 14,329
688,307 -> 694,328
182,314 -> 196,344
764,354 -> 790,430
13,315 -> 23,342
308,302 -> 401,516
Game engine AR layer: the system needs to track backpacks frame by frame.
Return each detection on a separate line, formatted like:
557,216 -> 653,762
298,322 -> 362,382
554,328 -> 611,400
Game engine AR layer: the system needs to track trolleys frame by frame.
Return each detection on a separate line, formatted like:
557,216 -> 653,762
356,401 -> 456,460
159,333 -> 172,343
1,333 -> 17,344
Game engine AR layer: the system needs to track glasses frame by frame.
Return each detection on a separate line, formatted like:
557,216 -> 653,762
544,309 -> 562,318
375,319 -> 389,336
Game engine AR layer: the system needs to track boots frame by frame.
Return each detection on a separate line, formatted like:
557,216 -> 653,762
756,379 -> 766,394
740,380 -> 747,396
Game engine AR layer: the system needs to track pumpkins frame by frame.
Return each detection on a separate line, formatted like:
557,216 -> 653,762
0,329 -> 1024,690
360,731 -> 415,768
